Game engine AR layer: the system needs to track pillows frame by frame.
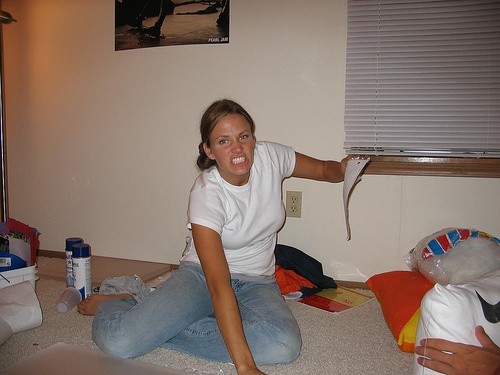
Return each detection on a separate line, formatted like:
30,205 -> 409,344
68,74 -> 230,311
363,271 -> 434,354
413,269 -> 500,375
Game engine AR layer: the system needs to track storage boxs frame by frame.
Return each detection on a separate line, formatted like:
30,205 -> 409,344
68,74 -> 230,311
0,262 -> 39,291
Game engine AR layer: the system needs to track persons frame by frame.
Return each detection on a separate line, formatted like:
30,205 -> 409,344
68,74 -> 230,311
76,99 -> 367,375
415,325 -> 500,375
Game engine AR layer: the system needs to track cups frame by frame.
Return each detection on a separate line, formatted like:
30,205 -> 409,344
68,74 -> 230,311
57,287 -> 82,312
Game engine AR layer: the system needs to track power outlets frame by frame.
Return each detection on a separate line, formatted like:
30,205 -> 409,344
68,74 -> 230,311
285,191 -> 302,218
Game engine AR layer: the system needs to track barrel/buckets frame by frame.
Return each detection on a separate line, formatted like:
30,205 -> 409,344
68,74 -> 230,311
0,263 -> 40,291
